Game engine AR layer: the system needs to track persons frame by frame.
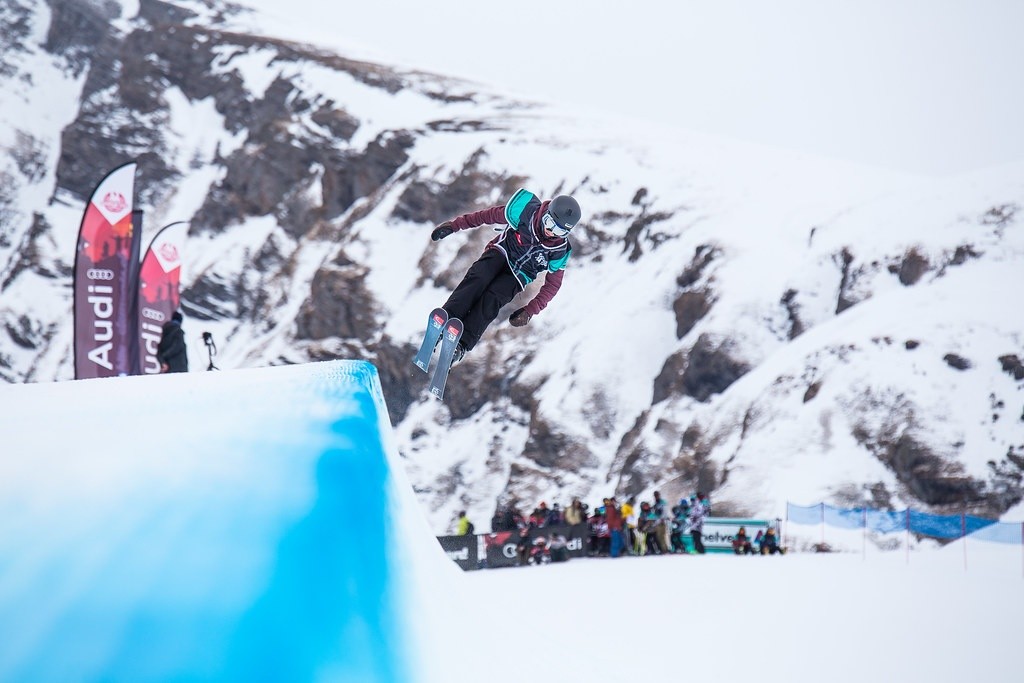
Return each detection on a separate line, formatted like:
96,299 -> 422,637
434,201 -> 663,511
454,491 -> 787,566
156,310 -> 189,374
432,188 -> 582,369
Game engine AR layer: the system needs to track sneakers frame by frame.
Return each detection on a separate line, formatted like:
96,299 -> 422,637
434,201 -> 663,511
434,329 -> 444,355
448,341 -> 468,374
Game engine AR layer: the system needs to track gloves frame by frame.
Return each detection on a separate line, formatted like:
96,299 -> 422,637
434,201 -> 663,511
508,307 -> 532,328
430,219 -> 453,241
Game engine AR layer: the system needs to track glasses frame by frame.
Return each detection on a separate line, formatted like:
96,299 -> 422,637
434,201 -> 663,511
542,213 -> 570,239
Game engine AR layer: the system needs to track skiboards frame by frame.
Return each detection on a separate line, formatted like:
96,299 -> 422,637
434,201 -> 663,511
412,308 -> 465,401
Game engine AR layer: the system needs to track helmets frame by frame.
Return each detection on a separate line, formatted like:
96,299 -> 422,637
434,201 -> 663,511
549,194 -> 583,231
